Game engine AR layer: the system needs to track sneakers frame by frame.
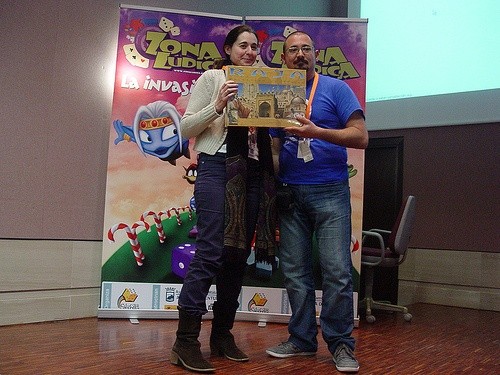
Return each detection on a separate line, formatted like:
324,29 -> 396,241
265,340 -> 317,359
332,343 -> 360,371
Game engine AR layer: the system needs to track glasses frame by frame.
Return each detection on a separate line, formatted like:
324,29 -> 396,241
284,47 -> 315,54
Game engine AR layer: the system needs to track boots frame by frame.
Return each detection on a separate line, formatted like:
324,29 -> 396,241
169,311 -> 218,372
210,310 -> 250,361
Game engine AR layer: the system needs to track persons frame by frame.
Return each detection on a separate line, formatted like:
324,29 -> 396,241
165,25 -> 258,371
265,31 -> 368,371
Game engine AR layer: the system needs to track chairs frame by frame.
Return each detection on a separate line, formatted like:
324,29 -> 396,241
358,194 -> 417,323
